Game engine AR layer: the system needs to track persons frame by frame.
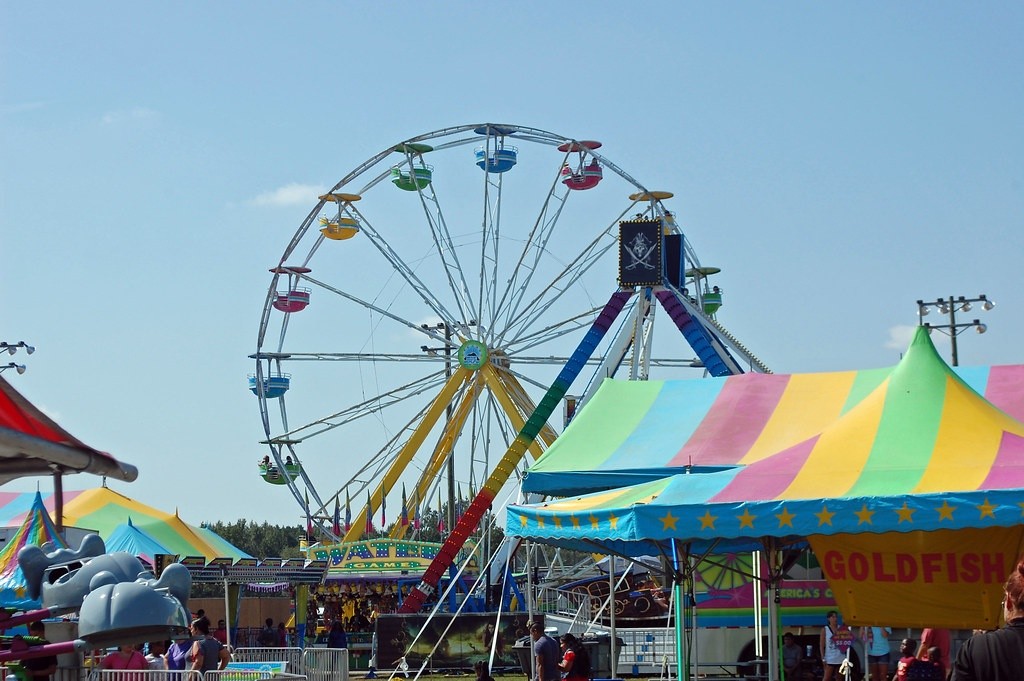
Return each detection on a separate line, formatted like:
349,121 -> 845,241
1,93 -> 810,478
589,157 -> 601,167
819,611 -> 849,681
557,633 -> 589,681
262,455 -> 270,468
213,619 -> 231,644
859,626 -> 893,681
165,630 -> 193,681
713,285 -> 719,293
777,631 -> 802,681
96,643 -> 150,681
921,646 -> 945,681
183,617 -> 230,681
482,621 -> 501,666
276,622 -> 287,651
950,559 -> 1024,681
257,618 -> 277,652
285,455 -> 294,466
636,580 -> 669,608
327,621 -> 347,680
18,621 -> 59,681
916,628 -> 951,681
145,640 -> 167,681
563,162 -> 574,176
349,608 -> 370,632
890,638 -> 920,681
528,621 -> 562,681
191,609 -> 204,622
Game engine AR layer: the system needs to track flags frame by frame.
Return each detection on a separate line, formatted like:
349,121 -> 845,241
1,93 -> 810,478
305,482 -> 481,541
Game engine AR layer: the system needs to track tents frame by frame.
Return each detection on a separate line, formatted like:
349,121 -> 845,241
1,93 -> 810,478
506,324 -> 1024,679
0,482 -> 256,613
